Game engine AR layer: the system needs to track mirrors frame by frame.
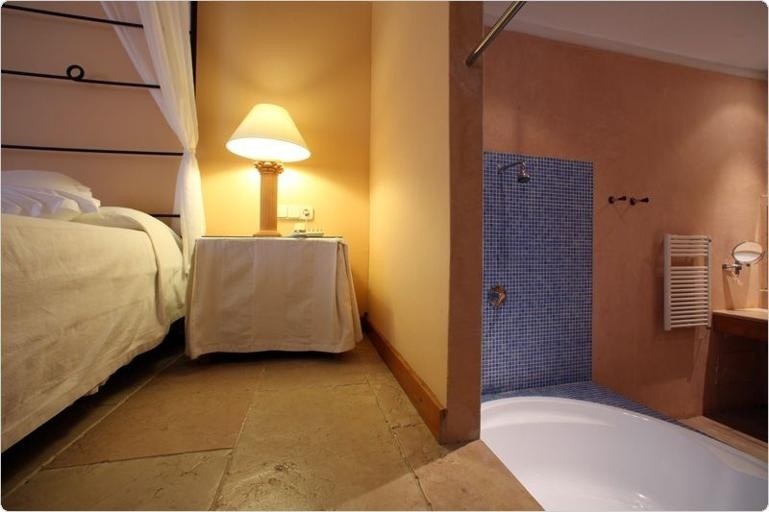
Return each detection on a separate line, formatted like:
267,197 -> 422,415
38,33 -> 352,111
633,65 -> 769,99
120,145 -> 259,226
731,240 -> 764,265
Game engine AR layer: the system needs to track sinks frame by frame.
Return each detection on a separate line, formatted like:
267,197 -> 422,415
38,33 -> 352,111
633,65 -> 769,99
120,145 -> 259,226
729,306 -> 768,320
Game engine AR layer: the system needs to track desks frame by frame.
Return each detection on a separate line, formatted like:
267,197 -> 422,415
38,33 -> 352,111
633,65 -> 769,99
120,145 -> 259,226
184,234 -> 365,362
711,307 -> 768,343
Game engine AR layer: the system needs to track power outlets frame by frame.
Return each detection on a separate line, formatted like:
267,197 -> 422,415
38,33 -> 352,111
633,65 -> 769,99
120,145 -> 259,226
299,205 -> 314,220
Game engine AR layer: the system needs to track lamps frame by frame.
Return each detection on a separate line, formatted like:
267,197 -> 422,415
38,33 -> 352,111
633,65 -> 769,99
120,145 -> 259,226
226,103 -> 312,237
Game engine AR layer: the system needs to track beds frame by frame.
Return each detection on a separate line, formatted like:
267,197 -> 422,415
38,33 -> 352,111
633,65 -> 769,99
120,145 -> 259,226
0,169 -> 189,454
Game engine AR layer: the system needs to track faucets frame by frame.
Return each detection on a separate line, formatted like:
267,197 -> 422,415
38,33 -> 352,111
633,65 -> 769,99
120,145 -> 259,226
489,287 -> 508,309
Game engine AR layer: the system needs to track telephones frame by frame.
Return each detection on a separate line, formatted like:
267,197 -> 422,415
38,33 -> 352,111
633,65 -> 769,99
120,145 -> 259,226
293,223 -> 324,238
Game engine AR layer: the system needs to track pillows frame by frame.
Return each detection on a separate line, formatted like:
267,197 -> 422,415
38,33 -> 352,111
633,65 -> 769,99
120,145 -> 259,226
72,207 -> 158,232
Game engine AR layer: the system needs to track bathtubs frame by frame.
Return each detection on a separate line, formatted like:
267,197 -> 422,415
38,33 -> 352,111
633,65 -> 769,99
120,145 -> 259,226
477,396 -> 769,511
